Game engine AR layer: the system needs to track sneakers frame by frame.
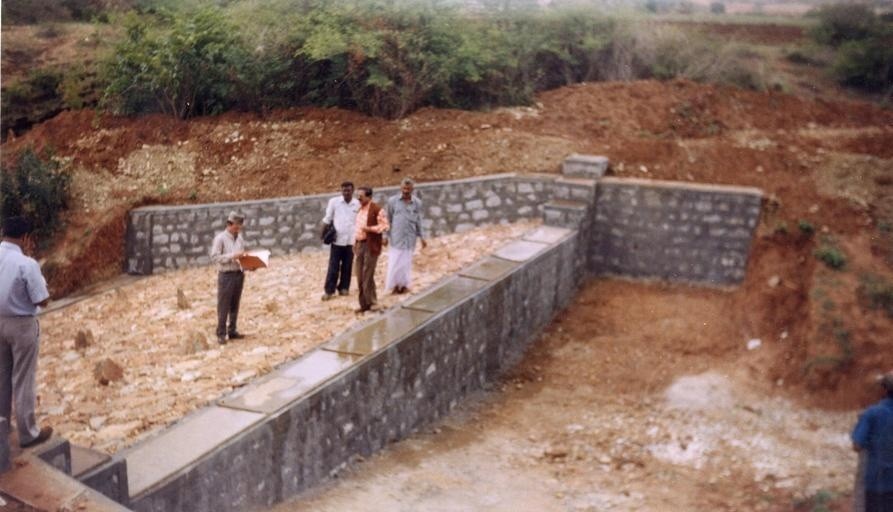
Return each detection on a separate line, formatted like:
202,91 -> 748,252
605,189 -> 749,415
339,288 -> 347,295
229,334 -> 243,339
20,426 -> 52,447
322,293 -> 332,300
218,335 -> 225,344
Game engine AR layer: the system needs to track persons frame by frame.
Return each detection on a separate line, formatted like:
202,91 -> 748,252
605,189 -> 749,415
317,181 -> 361,302
385,178 -> 427,295
350,185 -> 390,314
848,370 -> 892,511
0,216 -> 53,449
208,211 -> 248,344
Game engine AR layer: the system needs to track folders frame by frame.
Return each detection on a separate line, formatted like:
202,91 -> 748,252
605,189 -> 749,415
239,249 -> 271,270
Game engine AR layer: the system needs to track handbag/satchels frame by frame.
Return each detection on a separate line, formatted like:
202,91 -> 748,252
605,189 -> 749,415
322,224 -> 336,244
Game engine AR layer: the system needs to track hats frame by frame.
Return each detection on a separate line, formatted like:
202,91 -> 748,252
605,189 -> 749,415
227,211 -> 243,225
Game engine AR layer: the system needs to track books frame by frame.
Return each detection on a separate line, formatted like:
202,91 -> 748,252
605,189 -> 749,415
236,248 -> 270,272
